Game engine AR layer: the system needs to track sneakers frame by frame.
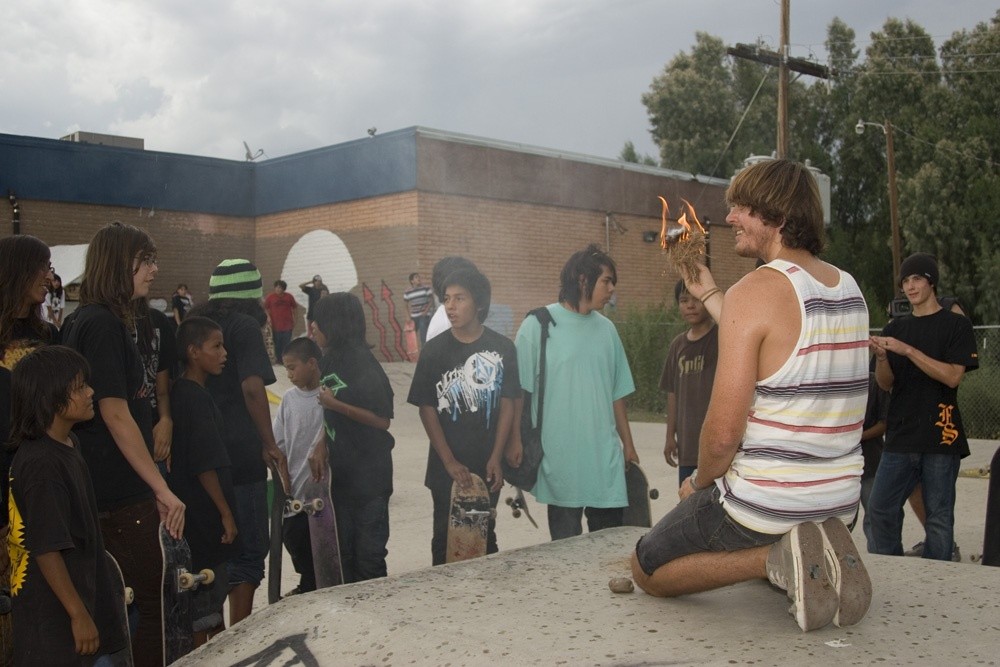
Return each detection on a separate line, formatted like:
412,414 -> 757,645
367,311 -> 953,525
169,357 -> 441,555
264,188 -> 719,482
904,540 -> 962,562
766,520 -> 840,632
821,515 -> 874,629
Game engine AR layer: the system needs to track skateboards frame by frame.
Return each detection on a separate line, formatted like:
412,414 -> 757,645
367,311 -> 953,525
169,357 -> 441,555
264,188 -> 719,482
620,461 -> 659,530
445,473 -> 498,564
505,467 -> 540,529
304,463 -> 346,590
94,549 -> 134,667
266,458 -> 302,605
158,519 -> 215,667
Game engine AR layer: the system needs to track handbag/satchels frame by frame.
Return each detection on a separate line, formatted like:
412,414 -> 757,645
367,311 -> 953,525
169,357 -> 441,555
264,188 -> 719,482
501,392 -> 545,492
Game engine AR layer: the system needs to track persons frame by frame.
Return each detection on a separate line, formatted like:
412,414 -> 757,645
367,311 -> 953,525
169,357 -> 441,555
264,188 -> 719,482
661,276 -> 722,501
851,252 -> 978,563
403,273 -> 438,349
0,236 -> 60,529
169,317 -> 238,650
504,244 -> 640,541
263,280 -> 298,364
407,257 -> 521,570
631,160 -> 872,631
172,284 -> 197,328
58,221 -> 186,667
299,274 -> 329,337
185,258 -> 291,640
7,345 -> 114,667
274,293 -> 394,598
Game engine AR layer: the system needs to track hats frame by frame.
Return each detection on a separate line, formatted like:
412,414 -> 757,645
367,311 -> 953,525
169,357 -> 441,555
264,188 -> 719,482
896,252 -> 938,290
208,258 -> 264,301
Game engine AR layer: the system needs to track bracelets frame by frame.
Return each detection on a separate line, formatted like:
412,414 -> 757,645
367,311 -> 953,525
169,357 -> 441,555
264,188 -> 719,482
690,468 -> 699,491
699,288 -> 717,302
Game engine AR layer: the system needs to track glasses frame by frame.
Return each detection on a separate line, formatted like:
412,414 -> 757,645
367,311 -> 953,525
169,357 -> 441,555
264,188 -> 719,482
39,263 -> 56,275
134,254 -> 159,268
